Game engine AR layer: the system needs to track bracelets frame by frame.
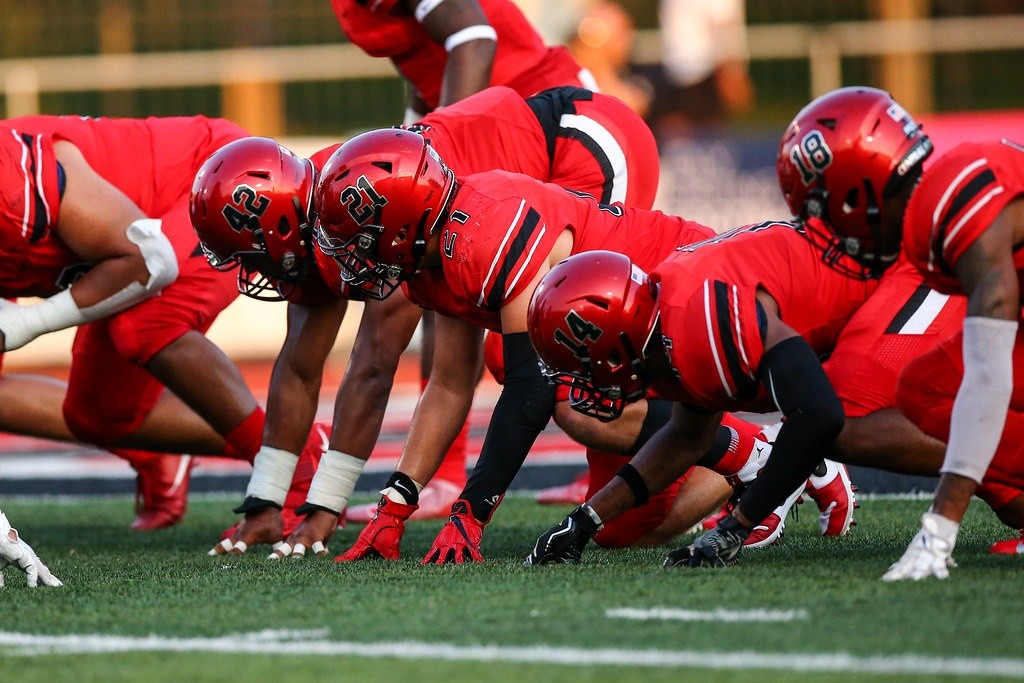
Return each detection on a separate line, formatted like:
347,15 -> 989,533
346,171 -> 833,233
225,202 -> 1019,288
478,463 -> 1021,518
616,462 -> 650,508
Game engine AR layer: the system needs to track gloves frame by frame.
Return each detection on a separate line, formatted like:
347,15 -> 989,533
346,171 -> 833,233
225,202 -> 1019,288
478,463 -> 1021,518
2,509 -> 64,590
881,511 -> 959,581
330,495 -> 421,564
522,502 -> 602,565
661,515 -> 748,569
0,297 -> 34,351
419,498 -> 504,566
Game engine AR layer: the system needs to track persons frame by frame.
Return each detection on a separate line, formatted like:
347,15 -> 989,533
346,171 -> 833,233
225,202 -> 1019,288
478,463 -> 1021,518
1,2 -> 1024,584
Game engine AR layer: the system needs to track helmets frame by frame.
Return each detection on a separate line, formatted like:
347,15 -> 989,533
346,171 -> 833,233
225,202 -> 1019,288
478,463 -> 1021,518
777,86 -> 934,281
314,128 -> 456,302
526,249 -> 661,422
189,136 -> 316,302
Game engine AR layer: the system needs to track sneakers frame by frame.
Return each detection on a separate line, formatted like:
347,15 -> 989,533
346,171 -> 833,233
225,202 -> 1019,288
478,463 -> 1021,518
308,422 -> 347,527
535,470 -> 592,506
133,454 -> 199,531
780,416 -> 861,538
741,442 -> 807,549
344,480 -> 465,519
